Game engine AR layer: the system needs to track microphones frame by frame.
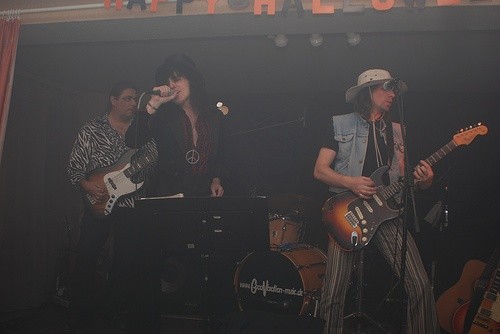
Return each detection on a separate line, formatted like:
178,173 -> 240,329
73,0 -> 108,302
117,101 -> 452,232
444,203 -> 449,228
146,88 -> 174,97
383,77 -> 399,91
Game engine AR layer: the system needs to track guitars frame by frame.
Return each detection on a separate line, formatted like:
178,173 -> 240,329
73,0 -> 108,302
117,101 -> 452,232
319,121 -> 489,255
79,101 -> 230,221
450,263 -> 500,334
435,260 -> 491,334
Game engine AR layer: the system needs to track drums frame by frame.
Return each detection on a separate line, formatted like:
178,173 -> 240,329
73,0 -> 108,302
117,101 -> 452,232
232,242 -> 328,316
268,209 -> 307,247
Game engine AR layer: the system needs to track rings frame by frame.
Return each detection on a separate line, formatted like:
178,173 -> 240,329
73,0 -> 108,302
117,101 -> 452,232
359,193 -> 363,198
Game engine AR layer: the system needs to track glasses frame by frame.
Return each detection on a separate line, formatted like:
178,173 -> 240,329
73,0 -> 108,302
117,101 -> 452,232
116,97 -> 138,102
382,80 -> 401,96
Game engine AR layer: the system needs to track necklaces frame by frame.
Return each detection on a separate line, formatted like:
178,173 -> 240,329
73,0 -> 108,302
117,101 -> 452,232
363,115 -> 387,137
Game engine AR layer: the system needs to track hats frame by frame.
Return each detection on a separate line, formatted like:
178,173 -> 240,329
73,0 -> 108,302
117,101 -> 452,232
345,69 -> 397,104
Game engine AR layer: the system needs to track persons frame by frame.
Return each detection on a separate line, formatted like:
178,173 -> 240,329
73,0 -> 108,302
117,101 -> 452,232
67,79 -> 155,334
125,54 -> 225,334
313,69 -> 442,334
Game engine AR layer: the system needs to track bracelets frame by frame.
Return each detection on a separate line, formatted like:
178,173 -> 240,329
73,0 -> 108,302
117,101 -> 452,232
147,102 -> 159,111
